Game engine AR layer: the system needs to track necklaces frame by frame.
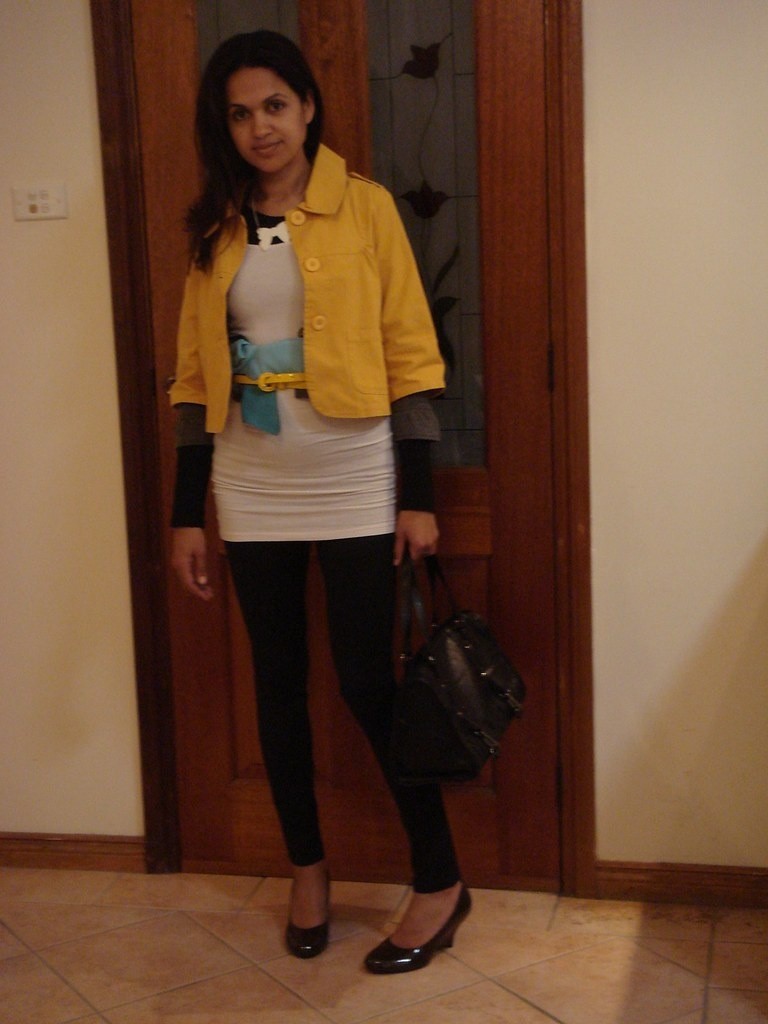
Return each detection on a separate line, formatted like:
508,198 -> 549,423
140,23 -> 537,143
250,187 -> 291,251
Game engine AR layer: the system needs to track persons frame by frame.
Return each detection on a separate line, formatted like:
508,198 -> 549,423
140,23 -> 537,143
166,29 -> 472,975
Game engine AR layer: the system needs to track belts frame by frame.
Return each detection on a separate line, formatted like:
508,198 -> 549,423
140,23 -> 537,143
233,372 -> 308,393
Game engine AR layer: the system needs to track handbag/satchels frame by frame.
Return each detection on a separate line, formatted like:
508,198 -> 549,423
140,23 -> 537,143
396,545 -> 525,777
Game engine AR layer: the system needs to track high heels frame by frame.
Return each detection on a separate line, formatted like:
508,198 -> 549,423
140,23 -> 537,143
365,885 -> 472,973
286,870 -> 330,958
